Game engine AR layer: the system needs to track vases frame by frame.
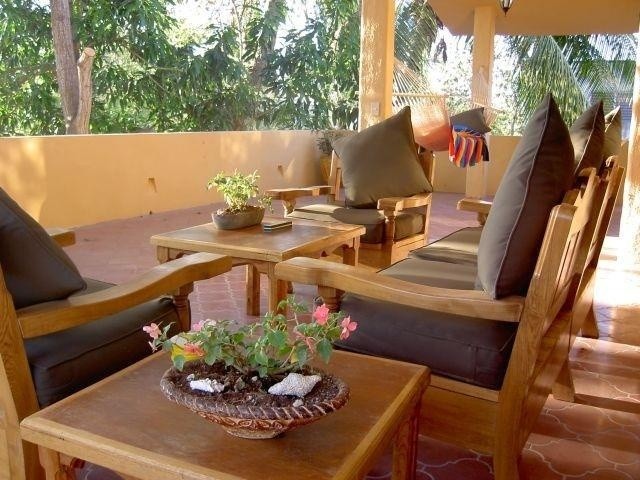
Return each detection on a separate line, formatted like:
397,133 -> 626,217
157,359 -> 351,441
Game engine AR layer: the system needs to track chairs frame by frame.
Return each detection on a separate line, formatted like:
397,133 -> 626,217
0,185 -> 234,476
269,147 -> 434,269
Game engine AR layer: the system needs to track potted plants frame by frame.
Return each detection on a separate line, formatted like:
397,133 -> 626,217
207,168 -> 272,228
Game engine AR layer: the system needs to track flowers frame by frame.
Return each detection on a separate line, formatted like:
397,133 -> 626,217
141,294 -> 357,378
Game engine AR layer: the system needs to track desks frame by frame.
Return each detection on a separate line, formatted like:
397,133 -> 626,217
20,342 -> 432,480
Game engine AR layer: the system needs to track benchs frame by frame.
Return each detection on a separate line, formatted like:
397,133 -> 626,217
275,154 -> 623,479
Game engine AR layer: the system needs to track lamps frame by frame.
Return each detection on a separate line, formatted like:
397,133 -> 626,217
499,0 -> 514,17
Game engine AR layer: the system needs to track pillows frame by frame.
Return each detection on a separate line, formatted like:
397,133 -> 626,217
477,94 -> 622,300
329,105 -> 432,207
1,189 -> 87,304
